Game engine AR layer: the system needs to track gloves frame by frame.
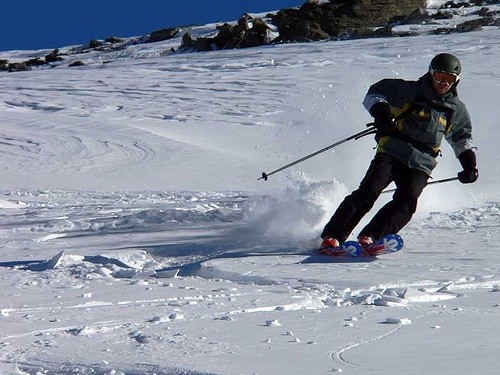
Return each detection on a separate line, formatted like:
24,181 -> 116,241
371,105 -> 397,132
458,150 -> 477,183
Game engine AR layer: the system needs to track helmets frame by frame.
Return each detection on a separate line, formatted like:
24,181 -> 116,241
429,54 -> 462,75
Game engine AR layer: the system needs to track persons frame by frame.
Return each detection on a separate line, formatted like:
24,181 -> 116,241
321,53 -> 478,250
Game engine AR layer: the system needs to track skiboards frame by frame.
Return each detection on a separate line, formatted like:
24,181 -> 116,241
319,234 -> 403,258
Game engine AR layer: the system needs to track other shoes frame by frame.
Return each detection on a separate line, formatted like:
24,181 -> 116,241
358,236 -> 373,247
319,235 -> 339,250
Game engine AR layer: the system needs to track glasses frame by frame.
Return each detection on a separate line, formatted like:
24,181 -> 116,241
429,66 -> 462,86
